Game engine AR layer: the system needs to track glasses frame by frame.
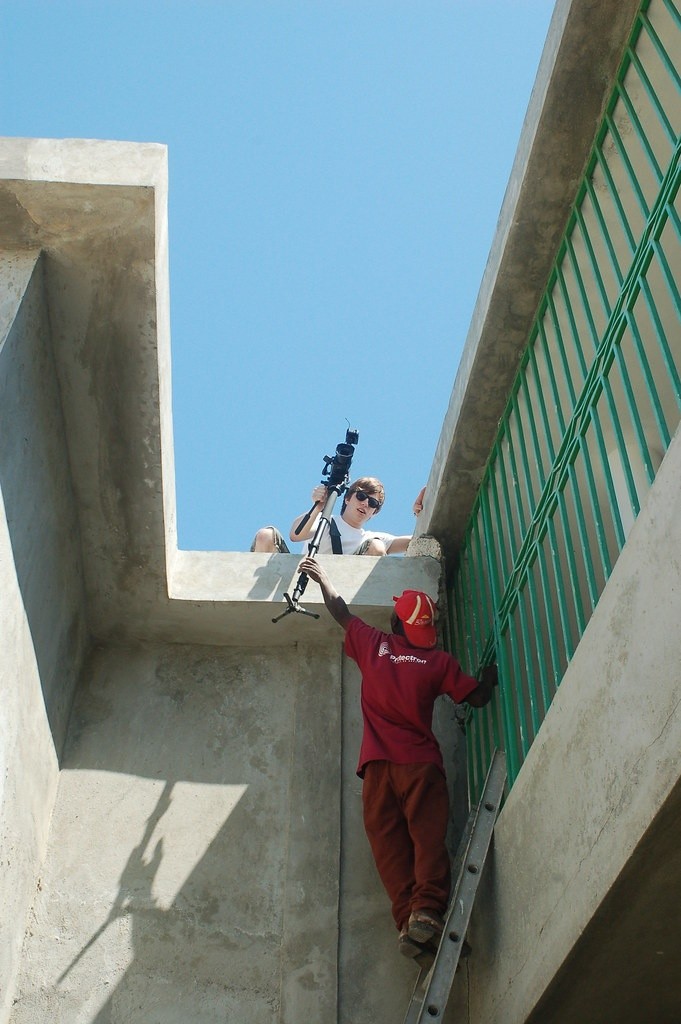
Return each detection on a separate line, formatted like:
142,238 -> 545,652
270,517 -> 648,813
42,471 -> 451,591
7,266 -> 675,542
354,490 -> 379,509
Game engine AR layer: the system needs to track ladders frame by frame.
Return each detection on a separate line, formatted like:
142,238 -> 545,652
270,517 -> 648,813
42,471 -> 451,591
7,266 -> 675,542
404,747 -> 507,1024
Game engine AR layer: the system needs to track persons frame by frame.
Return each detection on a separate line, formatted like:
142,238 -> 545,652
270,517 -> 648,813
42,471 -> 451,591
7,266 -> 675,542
249,476 -> 426,556
297,557 -> 498,972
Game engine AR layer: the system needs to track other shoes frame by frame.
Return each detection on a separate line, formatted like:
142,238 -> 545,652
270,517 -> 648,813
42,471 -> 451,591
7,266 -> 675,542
398,924 -> 461,975
407,911 -> 472,958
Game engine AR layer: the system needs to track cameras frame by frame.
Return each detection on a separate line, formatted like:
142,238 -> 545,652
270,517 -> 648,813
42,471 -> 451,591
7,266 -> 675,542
322,443 -> 355,492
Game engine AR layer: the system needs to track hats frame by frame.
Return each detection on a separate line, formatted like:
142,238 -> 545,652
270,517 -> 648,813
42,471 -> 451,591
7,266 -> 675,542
391,590 -> 438,648
